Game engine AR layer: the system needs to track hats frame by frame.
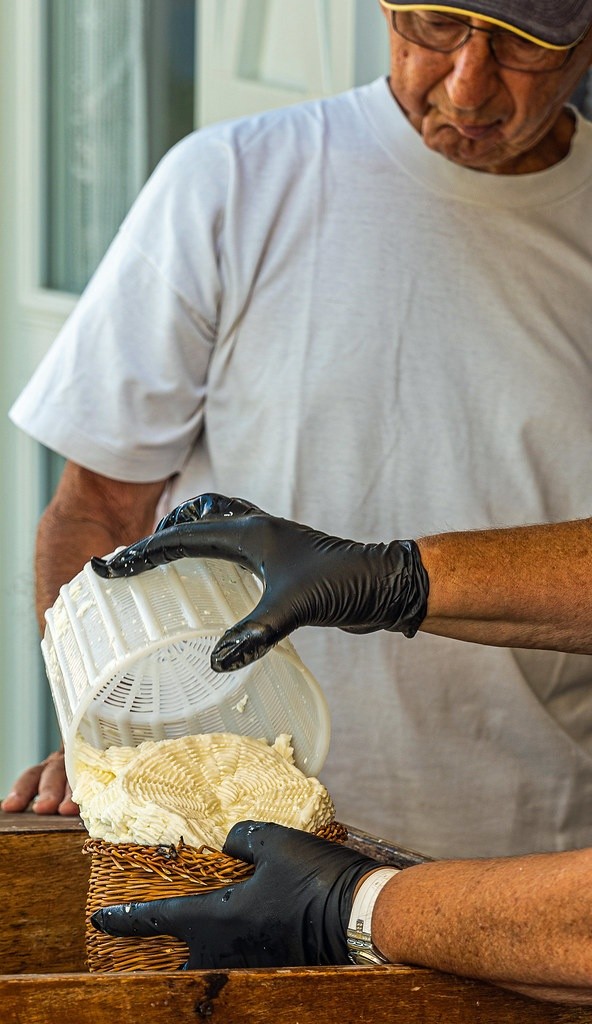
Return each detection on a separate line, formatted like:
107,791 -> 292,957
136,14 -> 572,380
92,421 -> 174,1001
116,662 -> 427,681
381,0 -> 592,50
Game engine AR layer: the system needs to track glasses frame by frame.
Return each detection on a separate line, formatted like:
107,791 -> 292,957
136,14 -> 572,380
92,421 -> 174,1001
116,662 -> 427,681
392,10 -> 576,73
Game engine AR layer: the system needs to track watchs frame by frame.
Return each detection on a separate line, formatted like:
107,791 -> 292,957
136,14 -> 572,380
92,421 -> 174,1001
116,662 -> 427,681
344,869 -> 402,964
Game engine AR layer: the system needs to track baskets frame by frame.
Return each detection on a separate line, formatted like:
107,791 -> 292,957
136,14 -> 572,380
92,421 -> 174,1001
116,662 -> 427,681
82,820 -> 348,974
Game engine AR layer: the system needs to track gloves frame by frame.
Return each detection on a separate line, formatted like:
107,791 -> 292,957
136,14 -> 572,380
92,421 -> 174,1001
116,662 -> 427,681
91,490 -> 432,675
91,821 -> 398,967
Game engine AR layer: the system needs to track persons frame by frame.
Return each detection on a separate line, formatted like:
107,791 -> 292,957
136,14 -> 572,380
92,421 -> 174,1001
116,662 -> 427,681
91,491 -> 592,1006
7,0 -> 592,858
